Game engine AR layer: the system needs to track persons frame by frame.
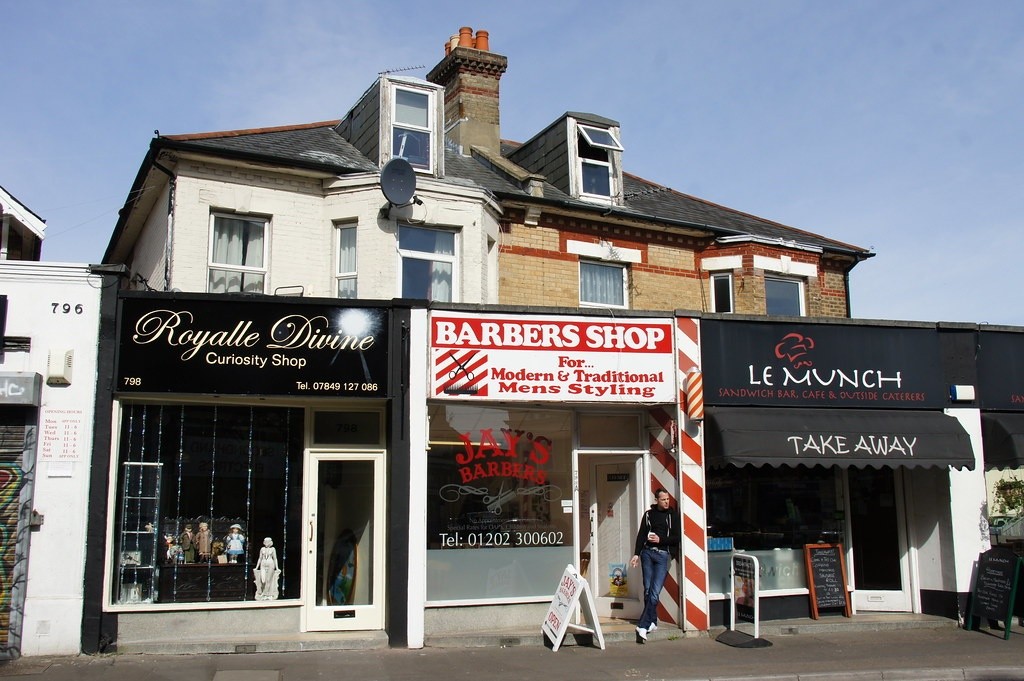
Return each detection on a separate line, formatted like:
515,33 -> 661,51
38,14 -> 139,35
253,538 -> 281,583
630,490 -> 677,640
180,523 -> 212,563
224,525 -> 245,563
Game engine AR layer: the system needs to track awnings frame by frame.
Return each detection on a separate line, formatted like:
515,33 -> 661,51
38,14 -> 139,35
981,412 -> 1024,472
704,407 -> 975,474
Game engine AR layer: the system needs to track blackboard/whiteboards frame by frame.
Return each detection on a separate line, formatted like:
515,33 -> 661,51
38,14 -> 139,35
969,548 -> 1024,621
802,543 -> 851,609
544,564 -> 604,638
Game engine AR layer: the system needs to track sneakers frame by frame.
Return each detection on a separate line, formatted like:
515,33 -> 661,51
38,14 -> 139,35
636,626 -> 647,640
647,622 -> 658,633
